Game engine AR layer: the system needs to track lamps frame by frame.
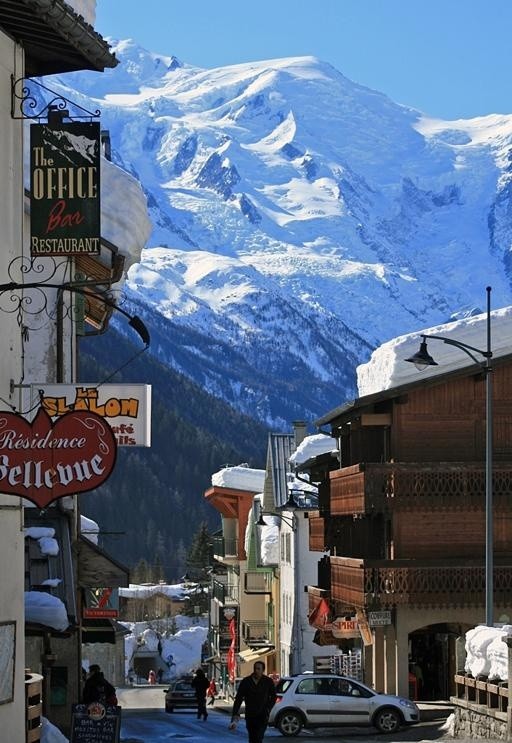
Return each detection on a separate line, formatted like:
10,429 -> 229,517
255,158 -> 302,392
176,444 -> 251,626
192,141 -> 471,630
253,511 -> 295,531
285,488 -> 332,512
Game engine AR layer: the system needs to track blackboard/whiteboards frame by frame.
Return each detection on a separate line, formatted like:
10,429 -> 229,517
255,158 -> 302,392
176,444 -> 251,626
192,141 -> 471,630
70,704 -> 121,743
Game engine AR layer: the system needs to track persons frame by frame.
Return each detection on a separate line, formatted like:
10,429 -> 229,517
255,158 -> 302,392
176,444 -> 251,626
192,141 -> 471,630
190,668 -> 210,721
229,659 -> 275,742
81,664 -> 119,704
208,677 -> 217,705
81,666 -> 87,701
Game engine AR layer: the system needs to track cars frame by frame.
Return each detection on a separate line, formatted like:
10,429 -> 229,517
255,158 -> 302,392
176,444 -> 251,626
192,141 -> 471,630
267,675 -> 421,736
163,676 -> 198,712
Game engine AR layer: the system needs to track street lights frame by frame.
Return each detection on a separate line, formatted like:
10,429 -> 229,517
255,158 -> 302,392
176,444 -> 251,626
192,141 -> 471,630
180,573 -> 212,681
404,286 -> 493,627
